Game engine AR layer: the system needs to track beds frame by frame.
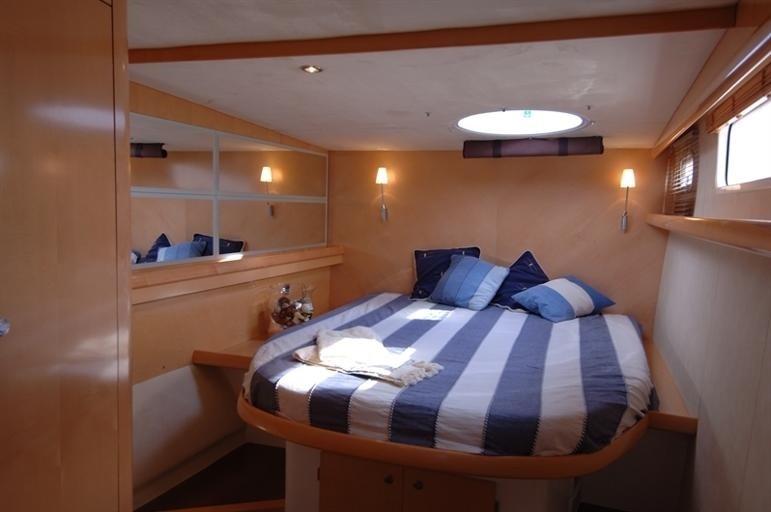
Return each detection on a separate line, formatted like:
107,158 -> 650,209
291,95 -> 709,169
236,289 -> 661,512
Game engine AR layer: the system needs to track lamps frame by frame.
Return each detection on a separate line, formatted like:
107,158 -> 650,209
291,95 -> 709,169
375,167 -> 390,224
259,166 -> 275,217
620,168 -> 636,234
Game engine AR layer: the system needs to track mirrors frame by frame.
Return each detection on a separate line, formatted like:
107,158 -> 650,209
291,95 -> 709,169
128,108 -> 330,272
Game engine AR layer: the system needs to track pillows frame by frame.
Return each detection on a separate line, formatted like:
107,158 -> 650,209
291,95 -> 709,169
408,246 -> 617,324
131,232 -> 245,264
